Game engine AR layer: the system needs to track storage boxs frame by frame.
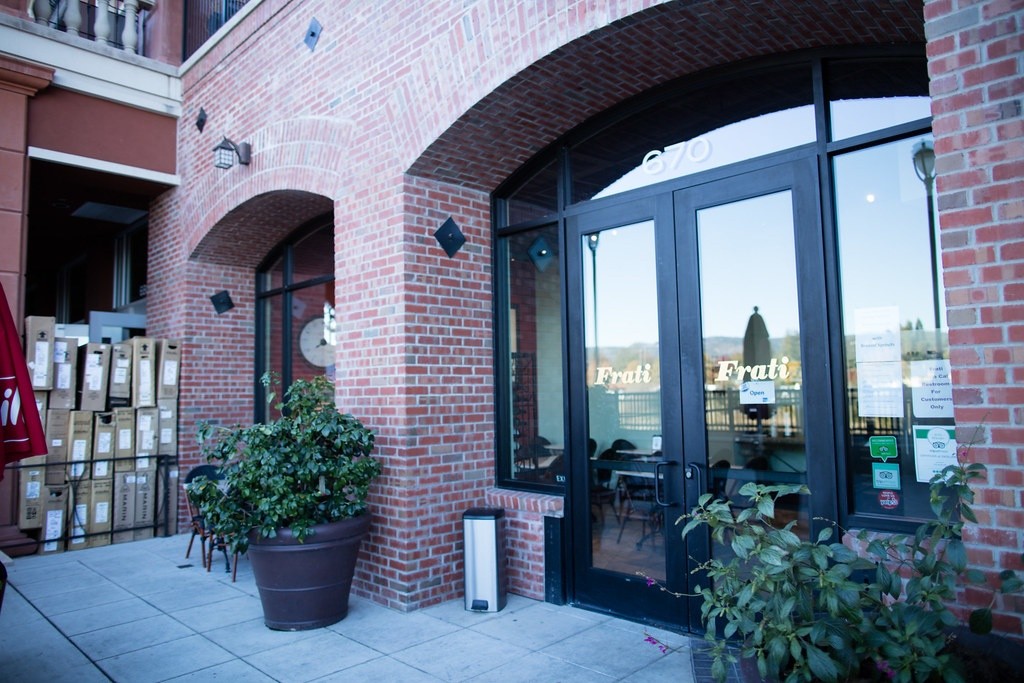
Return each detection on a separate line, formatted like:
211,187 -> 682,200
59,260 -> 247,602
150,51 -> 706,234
20,315 -> 182,557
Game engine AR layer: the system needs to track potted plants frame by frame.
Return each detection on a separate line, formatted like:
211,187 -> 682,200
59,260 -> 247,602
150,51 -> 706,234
192,369 -> 384,633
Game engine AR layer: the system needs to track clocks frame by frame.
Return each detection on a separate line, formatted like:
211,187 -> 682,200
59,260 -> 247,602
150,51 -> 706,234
299,316 -> 335,367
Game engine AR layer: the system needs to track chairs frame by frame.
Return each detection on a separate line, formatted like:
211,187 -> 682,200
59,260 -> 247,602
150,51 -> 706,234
610,466 -> 666,550
184,462 -> 240,582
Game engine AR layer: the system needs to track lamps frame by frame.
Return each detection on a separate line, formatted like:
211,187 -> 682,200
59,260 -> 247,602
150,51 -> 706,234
212,135 -> 251,170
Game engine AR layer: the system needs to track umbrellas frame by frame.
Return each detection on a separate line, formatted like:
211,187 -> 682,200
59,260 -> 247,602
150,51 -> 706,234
0,282 -> 48,484
737,306 -> 777,455
